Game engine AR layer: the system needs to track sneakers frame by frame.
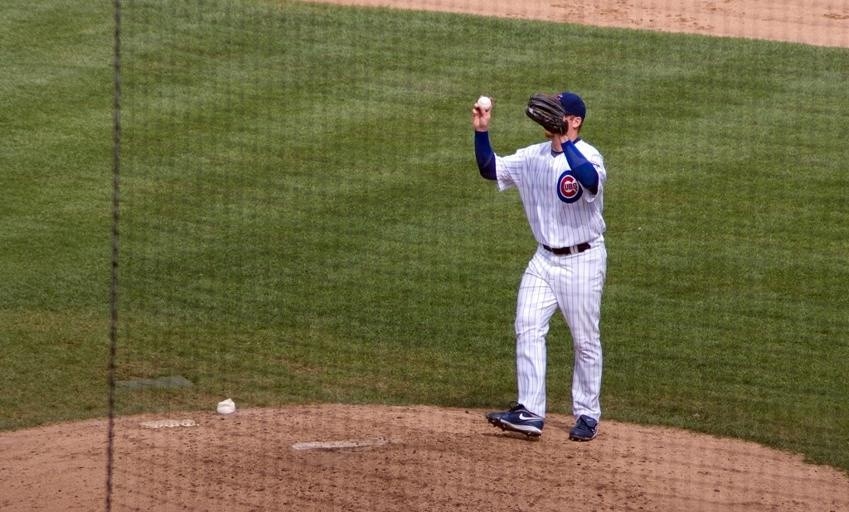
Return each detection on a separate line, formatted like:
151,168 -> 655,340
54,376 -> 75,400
569,415 -> 598,442
486,400 -> 545,439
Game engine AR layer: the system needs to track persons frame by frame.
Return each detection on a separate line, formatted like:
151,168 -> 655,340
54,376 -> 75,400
472,93 -> 608,442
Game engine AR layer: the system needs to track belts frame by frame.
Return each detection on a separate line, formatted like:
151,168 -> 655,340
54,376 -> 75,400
543,243 -> 590,254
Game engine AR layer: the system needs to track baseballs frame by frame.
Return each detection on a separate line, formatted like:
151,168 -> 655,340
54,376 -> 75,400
479,96 -> 491,110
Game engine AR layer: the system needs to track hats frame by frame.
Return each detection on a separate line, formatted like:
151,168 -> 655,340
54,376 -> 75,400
554,93 -> 586,119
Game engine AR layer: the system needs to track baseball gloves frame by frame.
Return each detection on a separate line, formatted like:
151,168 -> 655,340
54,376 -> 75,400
526,92 -> 569,135
